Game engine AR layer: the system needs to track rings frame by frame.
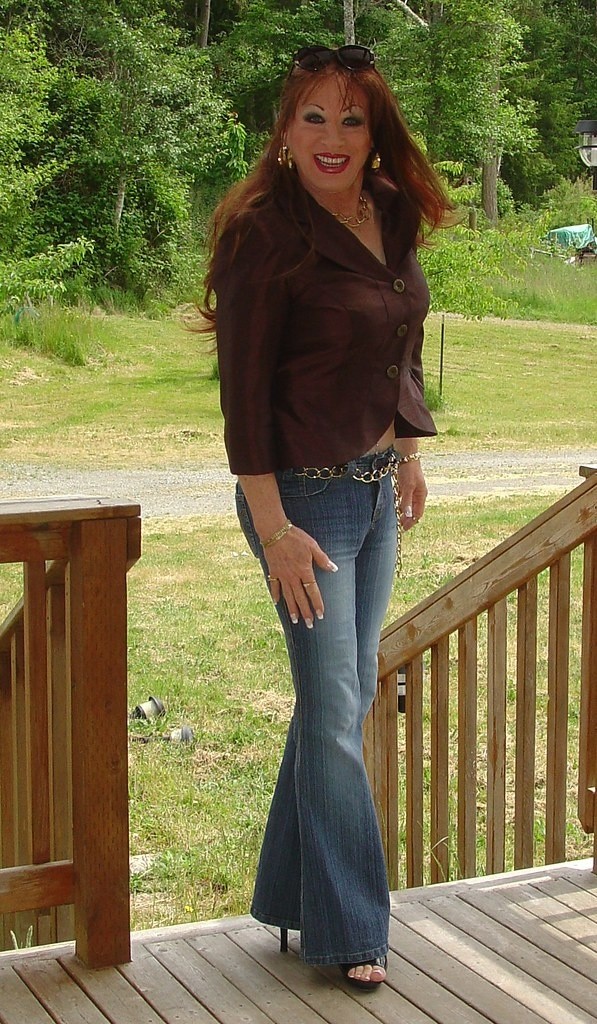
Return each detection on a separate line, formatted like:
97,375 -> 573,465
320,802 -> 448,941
302,581 -> 316,586
267,573 -> 278,581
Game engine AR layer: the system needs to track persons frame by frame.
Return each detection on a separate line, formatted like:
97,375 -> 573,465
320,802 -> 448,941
178,44 -> 469,992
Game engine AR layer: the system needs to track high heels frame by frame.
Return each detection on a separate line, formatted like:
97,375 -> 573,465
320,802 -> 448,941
279,927 -> 289,953
338,953 -> 388,990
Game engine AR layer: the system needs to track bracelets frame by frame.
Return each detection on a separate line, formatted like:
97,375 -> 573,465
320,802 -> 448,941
397,451 -> 421,463
258,519 -> 294,549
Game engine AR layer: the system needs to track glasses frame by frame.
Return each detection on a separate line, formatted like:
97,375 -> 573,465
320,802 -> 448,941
286,44 -> 375,84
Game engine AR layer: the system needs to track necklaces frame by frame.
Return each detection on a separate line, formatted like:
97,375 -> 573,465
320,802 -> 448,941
317,191 -> 373,226
